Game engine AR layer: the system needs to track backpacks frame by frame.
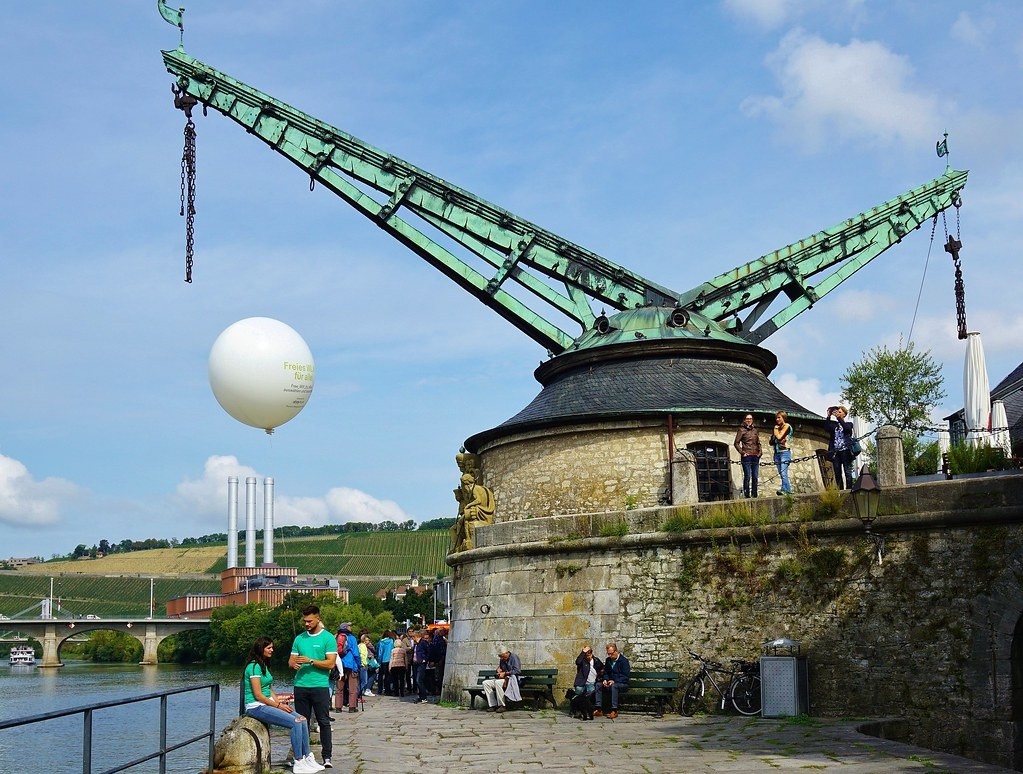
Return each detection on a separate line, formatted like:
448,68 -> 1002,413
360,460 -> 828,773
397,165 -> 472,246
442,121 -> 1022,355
337,633 -> 350,655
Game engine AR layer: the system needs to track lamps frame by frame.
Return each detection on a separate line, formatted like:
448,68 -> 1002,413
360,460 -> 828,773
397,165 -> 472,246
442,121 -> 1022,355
852,464 -> 885,556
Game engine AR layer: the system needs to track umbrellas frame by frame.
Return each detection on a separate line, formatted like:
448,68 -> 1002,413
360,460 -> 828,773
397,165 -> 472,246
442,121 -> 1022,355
961,330 -> 994,451
990,398 -> 1012,460
936,422 -> 950,475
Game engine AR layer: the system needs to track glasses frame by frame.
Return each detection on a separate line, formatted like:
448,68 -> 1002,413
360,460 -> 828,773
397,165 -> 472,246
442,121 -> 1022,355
608,650 -> 616,656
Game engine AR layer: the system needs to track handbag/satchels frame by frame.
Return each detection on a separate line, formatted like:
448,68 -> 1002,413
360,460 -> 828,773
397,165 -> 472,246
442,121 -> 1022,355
849,439 -> 862,456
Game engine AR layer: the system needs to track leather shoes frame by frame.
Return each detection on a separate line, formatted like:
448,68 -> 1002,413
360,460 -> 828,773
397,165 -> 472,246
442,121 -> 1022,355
593,710 -> 602,716
607,711 -> 618,718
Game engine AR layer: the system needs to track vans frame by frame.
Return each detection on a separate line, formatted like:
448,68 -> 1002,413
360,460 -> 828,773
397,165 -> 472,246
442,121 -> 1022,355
1,615 -> 10,620
86,615 -> 101,620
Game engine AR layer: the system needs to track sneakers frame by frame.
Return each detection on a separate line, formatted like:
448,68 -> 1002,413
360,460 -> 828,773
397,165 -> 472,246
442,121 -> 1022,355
364,690 -> 376,697
304,752 -> 325,770
293,754 -> 318,773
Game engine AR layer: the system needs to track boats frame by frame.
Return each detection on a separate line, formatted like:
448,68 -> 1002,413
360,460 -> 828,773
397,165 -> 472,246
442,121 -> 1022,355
9,645 -> 35,666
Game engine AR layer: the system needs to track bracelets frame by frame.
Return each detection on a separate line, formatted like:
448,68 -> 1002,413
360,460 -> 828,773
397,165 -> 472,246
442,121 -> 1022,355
276,702 -> 280,708
505,672 -> 506,676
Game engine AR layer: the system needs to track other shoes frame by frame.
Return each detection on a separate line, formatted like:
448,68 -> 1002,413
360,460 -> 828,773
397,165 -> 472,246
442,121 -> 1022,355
358,698 -> 367,703
496,705 -> 506,712
414,698 -> 427,704
349,707 -> 358,713
336,708 -> 342,713
776,491 -> 784,494
329,717 -> 334,721
487,706 -> 496,712
323,758 -> 332,768
316,726 -> 333,733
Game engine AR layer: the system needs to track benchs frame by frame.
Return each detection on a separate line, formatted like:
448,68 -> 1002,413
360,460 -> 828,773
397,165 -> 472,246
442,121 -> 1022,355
618,671 -> 680,719
941,447 -> 1004,476
461,668 -> 559,710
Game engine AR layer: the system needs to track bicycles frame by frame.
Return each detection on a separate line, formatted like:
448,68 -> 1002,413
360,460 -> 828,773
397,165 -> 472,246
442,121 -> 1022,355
681,650 -> 763,717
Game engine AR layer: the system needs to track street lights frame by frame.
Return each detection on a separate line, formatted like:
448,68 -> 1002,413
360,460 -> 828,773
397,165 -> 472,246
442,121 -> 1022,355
406,584 -> 411,590
424,583 -> 429,590
447,581 -> 452,624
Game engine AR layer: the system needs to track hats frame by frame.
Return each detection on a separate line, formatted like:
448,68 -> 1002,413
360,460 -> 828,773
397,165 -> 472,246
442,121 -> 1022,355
340,622 -> 351,629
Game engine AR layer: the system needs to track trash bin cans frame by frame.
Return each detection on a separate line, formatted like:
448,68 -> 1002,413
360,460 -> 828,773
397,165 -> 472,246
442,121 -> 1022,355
759,638 -> 809,718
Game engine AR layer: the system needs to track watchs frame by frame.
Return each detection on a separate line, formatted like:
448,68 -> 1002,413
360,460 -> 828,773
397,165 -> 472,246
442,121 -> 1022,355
310,658 -> 314,665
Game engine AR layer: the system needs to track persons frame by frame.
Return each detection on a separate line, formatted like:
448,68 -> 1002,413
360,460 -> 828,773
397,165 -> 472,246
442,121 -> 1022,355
243,637 -> 325,773
482,644 -> 521,713
573,646 -> 605,721
593,643 -> 630,720
287,606 -> 337,767
769,410 -> 792,496
825,405 -> 854,490
447,473 -> 495,555
333,621 -> 447,713
734,414 -> 762,498
